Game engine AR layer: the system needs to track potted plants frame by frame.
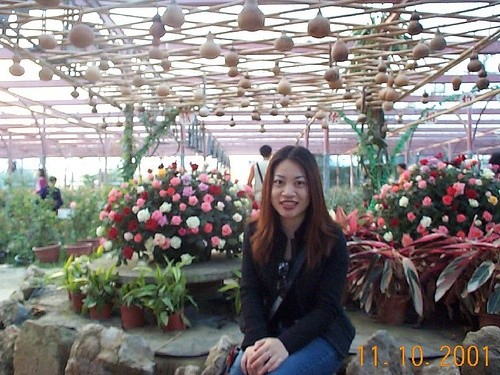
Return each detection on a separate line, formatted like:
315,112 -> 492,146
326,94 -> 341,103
330,203 -> 500,332
64,248 -> 200,330
33,177 -> 111,262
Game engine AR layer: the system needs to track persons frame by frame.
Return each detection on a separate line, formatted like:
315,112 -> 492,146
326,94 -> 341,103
228,145 -> 357,375
36,168 -> 47,193
233,179 -> 239,188
40,176 -> 63,215
397,163 -> 406,181
247,145 -> 273,203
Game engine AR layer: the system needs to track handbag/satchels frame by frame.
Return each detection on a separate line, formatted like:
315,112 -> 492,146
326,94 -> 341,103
199,335 -> 241,375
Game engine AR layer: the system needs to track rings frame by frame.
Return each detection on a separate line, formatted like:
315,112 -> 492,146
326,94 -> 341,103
267,352 -> 272,357
